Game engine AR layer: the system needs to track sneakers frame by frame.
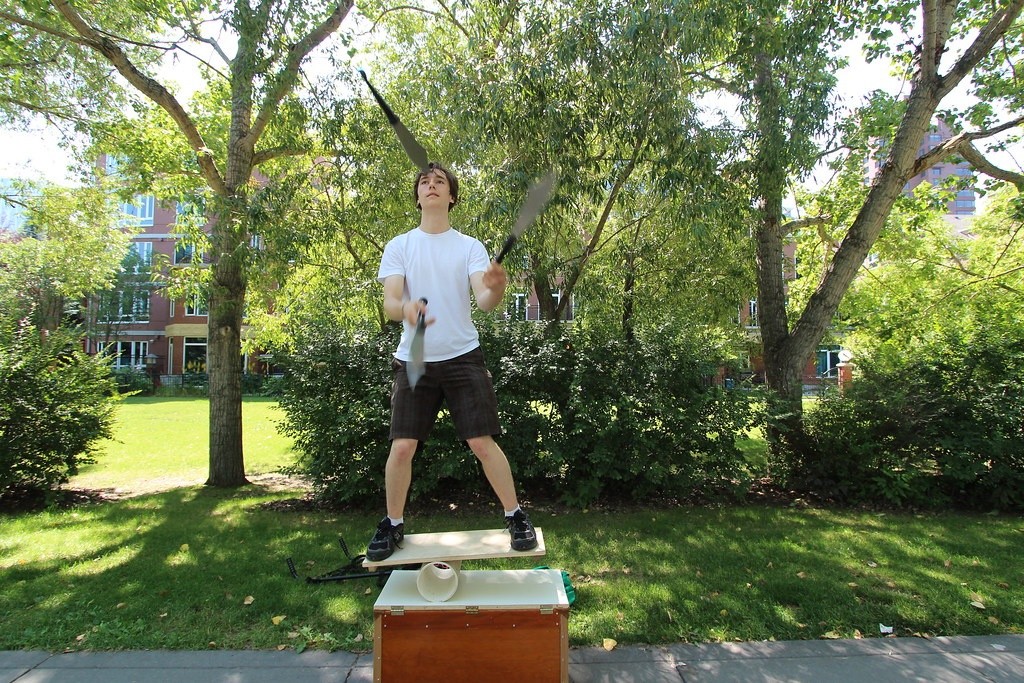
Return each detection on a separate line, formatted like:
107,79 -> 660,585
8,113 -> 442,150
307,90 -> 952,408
366,515 -> 404,561
503,509 -> 537,550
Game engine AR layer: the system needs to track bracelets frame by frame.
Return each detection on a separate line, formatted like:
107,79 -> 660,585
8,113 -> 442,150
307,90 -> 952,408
400,301 -> 406,321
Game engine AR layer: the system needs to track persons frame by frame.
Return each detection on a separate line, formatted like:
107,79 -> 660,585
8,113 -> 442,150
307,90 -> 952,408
363,165 -> 541,562
